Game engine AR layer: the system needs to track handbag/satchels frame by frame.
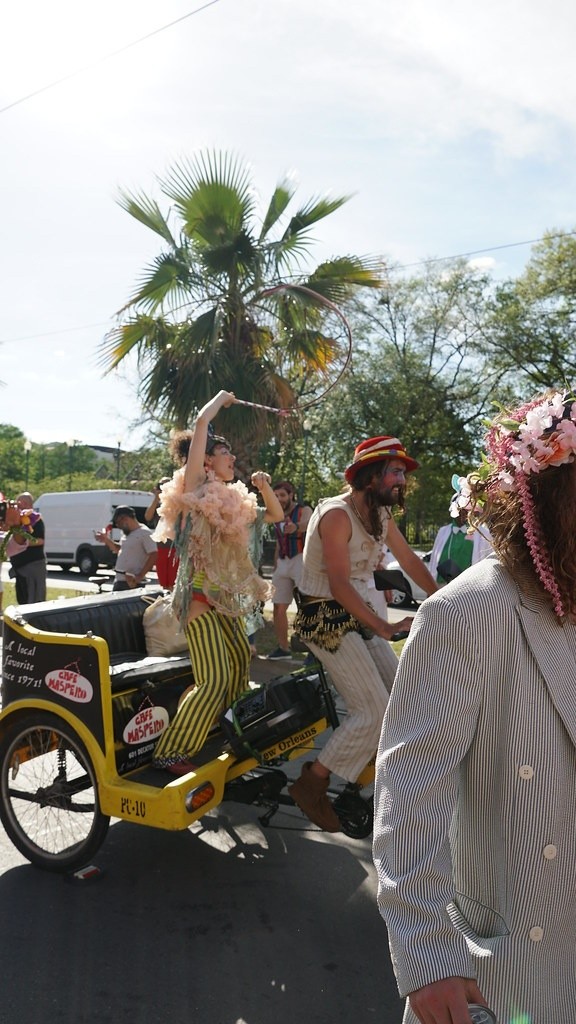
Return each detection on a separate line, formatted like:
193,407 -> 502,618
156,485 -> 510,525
141,594 -> 189,657
437,559 -> 463,584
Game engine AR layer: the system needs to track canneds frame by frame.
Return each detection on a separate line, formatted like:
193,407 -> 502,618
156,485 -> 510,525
466,1004 -> 498,1024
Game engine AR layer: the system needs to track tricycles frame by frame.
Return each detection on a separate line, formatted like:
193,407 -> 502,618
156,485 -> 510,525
0,583 -> 409,873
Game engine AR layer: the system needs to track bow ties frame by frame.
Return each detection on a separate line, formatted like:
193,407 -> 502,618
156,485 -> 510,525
453,525 -> 467,534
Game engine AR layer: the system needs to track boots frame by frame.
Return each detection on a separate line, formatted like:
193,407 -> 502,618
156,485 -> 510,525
289,761 -> 342,833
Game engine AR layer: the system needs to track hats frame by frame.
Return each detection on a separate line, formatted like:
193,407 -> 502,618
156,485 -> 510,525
110,506 -> 135,521
273,482 -> 293,493
451,474 -> 484,513
345,437 -> 419,485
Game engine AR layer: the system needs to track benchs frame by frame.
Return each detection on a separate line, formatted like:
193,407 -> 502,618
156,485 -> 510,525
4,584 -> 192,695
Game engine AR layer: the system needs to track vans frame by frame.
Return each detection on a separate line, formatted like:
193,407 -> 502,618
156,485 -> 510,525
30,489 -> 155,578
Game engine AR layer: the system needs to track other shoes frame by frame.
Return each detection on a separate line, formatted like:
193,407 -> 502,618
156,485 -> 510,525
167,760 -> 198,775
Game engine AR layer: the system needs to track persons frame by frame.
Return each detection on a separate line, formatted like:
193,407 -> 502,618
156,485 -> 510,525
93,504 -> 157,591
10,492 -> 46,604
428,493 -> 492,583
288,436 -> 438,833
372,389 -> 576,1024
151,390 -> 285,774
268,481 -> 313,657
0,509 -> 41,558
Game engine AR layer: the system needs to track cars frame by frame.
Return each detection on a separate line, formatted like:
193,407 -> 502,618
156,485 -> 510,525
382,550 -> 431,608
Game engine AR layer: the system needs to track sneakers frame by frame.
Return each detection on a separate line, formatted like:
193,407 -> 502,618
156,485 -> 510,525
266,645 -> 293,660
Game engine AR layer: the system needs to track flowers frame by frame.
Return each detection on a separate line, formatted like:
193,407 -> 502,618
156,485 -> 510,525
449,378 -> 576,541
